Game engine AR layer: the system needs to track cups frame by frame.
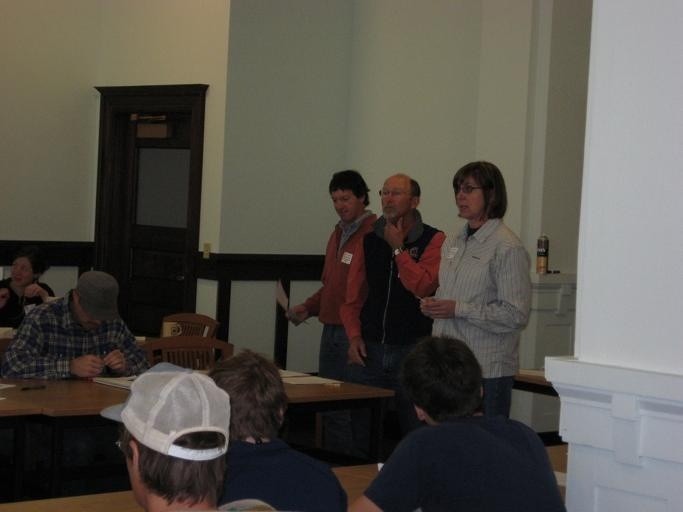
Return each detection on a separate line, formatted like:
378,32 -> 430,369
162,322 -> 182,336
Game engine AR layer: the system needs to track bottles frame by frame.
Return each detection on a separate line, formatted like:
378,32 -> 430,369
537,235 -> 548,275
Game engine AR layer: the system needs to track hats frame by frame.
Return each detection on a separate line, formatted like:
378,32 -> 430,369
76,271 -> 121,320
99,362 -> 231,461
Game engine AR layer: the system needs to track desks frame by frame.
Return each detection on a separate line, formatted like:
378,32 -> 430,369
0,370 -> 395,464
2,444 -> 568,512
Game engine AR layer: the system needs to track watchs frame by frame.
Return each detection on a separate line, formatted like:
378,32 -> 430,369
389,246 -> 407,258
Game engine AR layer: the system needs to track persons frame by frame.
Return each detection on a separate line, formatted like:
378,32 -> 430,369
0,246 -> 56,329
337,174 -> 447,462
208,349 -> 348,512
283,170 -> 372,454
348,333 -> 565,512
419,162 -> 533,420
0,270 -> 147,378
99,361 -> 279,512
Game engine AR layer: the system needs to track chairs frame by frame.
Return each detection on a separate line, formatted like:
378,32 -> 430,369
162,313 -> 220,343
143,335 -> 233,373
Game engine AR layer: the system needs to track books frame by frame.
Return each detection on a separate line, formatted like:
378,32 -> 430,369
92,374 -> 137,391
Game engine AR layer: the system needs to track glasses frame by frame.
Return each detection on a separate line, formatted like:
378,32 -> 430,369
455,185 -> 481,193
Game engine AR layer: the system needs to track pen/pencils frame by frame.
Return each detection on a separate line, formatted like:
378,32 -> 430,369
21,386 -> 46,391
